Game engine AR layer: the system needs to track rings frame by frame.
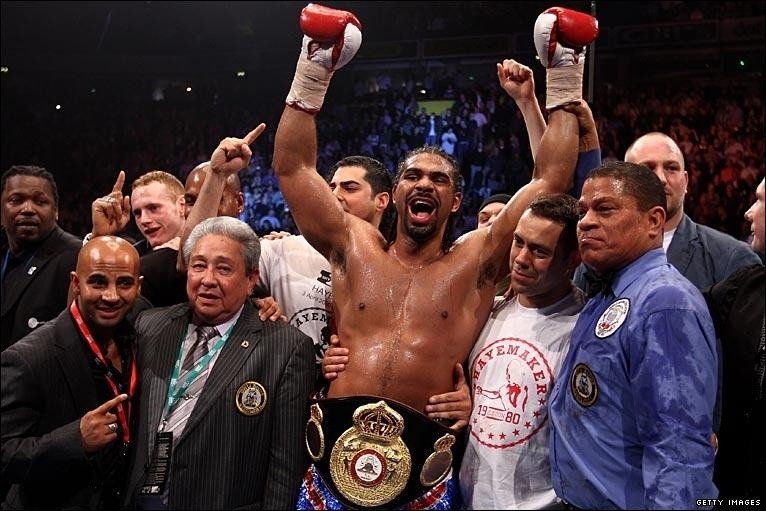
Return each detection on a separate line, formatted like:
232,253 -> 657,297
108,421 -> 116,430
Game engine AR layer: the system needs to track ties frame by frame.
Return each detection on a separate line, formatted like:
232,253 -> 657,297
157,326 -> 219,452
105,337 -> 123,372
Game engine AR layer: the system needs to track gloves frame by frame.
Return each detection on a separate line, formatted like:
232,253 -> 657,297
284,3 -> 363,115
532,5 -> 600,111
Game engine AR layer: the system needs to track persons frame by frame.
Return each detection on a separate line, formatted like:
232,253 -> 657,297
742,177 -> 766,255
546,98 -> 718,510
603,87 -> 765,177
623,130 -> 766,511
458,193 -> 590,509
126,169 -> 187,256
474,59 -> 547,228
273,1 -> 599,510
1,236 -> 287,508
325,74 -> 471,166
0,165 -> 83,351
176,124 -> 392,392
83,161 -> 246,308
129,215 -> 314,510
